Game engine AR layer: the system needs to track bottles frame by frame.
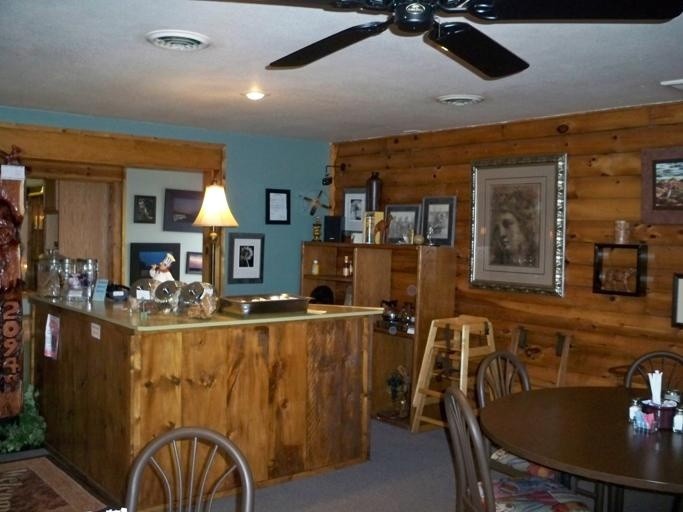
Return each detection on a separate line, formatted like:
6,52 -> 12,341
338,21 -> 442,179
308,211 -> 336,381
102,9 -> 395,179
35,248 -> 98,301
341,254 -> 353,278
627,398 -> 641,423
364,170 -> 383,210
126,278 -> 221,321
310,259 -> 320,275
665,389 -> 681,406
671,407 -> 682,434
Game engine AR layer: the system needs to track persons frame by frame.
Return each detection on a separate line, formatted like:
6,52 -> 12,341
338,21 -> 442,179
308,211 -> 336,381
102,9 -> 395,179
489,183 -> 538,268
149,252 -> 176,283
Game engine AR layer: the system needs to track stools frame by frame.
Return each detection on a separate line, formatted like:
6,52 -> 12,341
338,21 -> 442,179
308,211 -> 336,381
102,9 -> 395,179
410,314 -> 502,433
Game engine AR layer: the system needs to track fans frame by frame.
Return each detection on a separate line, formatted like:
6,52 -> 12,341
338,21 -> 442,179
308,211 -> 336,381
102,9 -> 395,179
299,189 -> 332,242
212,0 -> 683,78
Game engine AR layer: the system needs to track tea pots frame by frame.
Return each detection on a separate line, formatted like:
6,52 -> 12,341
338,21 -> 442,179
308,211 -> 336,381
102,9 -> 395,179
375,298 -> 416,324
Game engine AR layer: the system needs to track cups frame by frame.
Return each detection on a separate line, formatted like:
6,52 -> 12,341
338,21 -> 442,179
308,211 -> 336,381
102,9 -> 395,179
613,218 -> 631,245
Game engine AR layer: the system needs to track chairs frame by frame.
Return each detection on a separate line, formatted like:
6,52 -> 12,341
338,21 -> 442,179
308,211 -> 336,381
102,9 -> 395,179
475,350 -> 562,482
623,351 -> 682,390
125,424 -> 258,511
442,386 -> 592,512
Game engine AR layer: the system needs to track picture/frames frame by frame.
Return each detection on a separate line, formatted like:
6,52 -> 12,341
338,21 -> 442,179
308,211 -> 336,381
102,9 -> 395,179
421,195 -> 457,247
383,204 -> 422,242
641,146 -> 683,226
162,188 -> 205,232
186,251 -> 203,274
265,188 -> 291,225
133,194 -> 157,224
227,232 -> 265,284
671,272 -> 683,329
130,242 -> 181,289
342,186 -> 370,235
466,153 -> 568,298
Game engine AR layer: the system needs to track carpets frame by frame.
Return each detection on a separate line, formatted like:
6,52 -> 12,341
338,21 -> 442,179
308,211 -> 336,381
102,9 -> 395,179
0,446 -> 126,511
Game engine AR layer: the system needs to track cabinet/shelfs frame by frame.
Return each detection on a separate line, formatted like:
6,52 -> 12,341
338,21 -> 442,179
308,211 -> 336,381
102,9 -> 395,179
299,239 -> 457,430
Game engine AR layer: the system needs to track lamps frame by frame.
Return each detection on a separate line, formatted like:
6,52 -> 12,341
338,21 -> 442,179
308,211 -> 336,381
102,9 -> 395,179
322,162 -> 346,186
240,81 -> 272,101
193,183 -> 239,312
